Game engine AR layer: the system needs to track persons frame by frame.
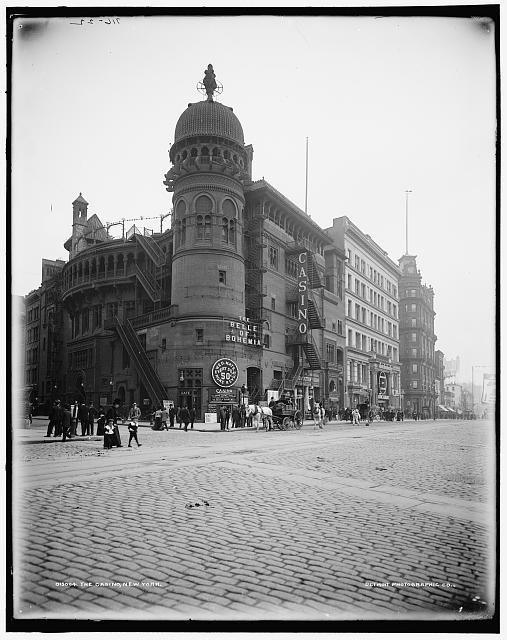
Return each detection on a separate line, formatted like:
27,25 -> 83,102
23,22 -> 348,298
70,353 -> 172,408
28,397 -> 198,449
404,409 -> 478,421
218,394 -> 404,432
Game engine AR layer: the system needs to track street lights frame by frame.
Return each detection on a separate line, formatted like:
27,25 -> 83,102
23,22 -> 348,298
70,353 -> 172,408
241,384 -> 248,428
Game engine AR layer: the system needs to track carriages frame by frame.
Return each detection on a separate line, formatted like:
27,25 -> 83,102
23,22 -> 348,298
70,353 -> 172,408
312,400 -> 334,429
356,404 -> 376,423
245,403 -> 304,432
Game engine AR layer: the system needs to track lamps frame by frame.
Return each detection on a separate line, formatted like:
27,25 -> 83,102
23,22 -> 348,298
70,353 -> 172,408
109,375 -> 114,386
80,378 -> 83,387
